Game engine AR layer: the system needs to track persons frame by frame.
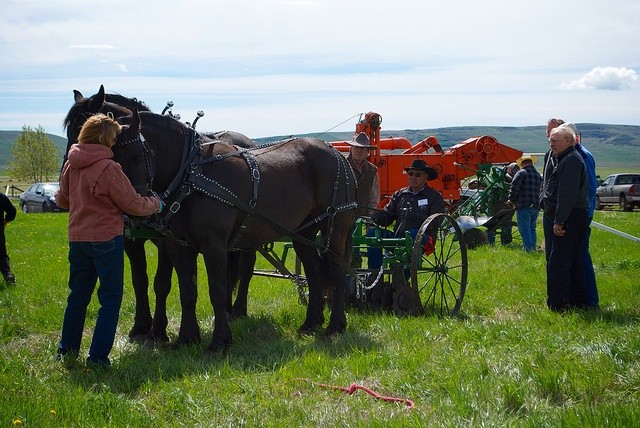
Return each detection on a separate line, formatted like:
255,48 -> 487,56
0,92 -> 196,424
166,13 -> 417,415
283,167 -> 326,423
344,132 -> 380,215
545,126 -> 590,313
367,160 -> 445,282
55,113 -> 163,370
0,192 -> 17,284
543,118 -> 565,264
558,122 -> 599,307
507,156 -> 541,253
488,162 -> 520,246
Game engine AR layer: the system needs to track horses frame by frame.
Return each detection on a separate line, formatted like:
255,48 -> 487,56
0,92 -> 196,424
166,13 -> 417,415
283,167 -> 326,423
110,110 -> 363,350
58,83 -> 260,347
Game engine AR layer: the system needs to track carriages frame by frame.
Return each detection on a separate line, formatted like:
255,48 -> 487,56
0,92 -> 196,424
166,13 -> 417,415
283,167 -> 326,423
63,82 -> 470,360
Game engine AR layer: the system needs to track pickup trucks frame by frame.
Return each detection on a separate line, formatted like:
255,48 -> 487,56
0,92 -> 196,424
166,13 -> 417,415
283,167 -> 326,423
592,172 -> 640,211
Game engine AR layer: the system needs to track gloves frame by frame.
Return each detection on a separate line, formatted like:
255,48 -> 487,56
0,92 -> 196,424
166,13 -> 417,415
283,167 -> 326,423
374,212 -> 391,225
402,208 -> 418,224
157,196 -> 163,213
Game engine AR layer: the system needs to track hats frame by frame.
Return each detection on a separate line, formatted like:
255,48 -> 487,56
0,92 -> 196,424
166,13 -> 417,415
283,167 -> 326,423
345,131 -> 378,149
517,154 -> 538,167
510,162 -> 518,174
404,159 -> 438,181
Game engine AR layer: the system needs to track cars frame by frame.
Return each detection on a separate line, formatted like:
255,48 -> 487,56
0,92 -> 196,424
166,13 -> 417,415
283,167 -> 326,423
19,182 -> 63,213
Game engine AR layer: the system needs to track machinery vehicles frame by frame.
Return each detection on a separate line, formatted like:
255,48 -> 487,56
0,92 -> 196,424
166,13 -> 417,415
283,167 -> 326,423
325,110 -> 524,221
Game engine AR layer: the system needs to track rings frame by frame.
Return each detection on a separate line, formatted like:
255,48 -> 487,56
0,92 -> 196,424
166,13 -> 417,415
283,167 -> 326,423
557,231 -> 559,234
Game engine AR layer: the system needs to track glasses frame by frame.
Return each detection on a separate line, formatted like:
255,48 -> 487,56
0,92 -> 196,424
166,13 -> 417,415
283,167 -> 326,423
110,137 -> 118,142
548,139 -> 560,143
407,170 -> 426,177
